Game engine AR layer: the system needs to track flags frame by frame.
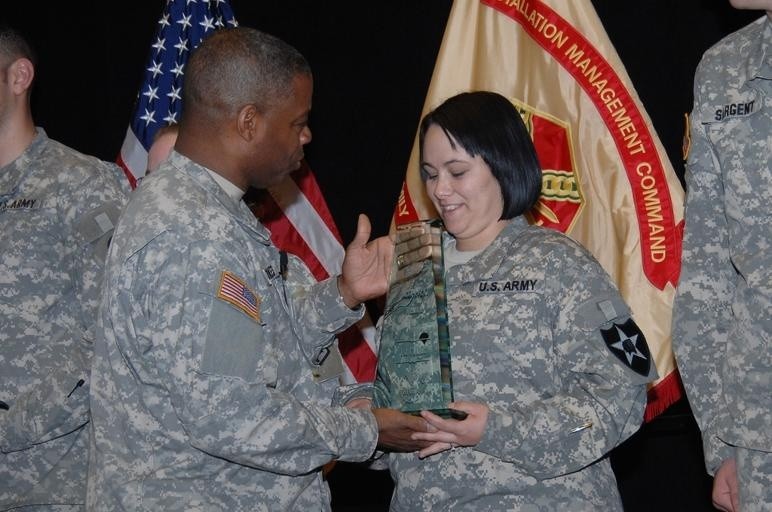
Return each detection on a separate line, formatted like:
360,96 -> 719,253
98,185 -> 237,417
112,1 -> 381,382
385,0 -> 687,425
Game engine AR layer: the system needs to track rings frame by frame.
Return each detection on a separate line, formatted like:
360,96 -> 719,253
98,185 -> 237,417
449,442 -> 456,454
396,254 -> 405,268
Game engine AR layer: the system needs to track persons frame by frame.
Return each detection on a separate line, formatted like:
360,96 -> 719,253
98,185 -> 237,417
329,89 -> 654,510
79,26 -> 447,511
669,0 -> 772,511
138,123 -> 347,479
0,23 -> 130,509
